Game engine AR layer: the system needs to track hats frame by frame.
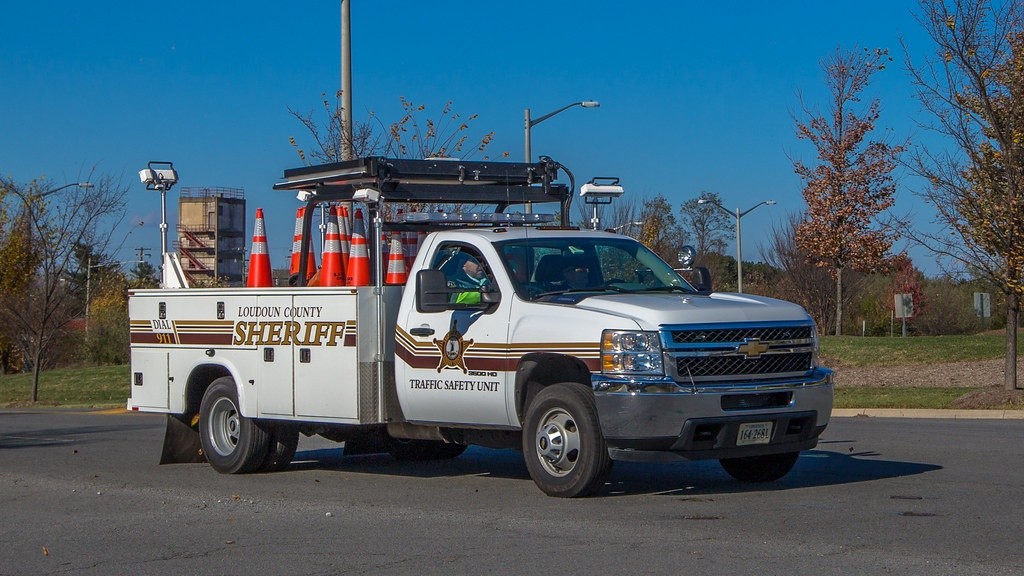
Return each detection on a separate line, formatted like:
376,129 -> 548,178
458,250 -> 482,268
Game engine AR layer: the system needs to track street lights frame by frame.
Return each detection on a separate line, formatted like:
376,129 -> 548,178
28,184 -> 96,353
84,260 -> 146,341
525,100 -> 601,228
699,199 -> 777,294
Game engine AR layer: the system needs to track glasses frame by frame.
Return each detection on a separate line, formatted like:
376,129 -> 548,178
467,256 -> 487,266
570,266 -> 589,274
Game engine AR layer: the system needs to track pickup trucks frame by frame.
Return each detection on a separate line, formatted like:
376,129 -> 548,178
127,157 -> 836,497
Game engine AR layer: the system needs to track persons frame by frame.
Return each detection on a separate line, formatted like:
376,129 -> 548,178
549,252 -> 598,291
449,246 -> 493,304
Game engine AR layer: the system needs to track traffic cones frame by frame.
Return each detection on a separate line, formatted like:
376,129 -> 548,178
291,207 -> 316,280
319,206 -> 352,287
246,206 -> 273,288
368,208 -> 427,284
345,208 -> 371,287
